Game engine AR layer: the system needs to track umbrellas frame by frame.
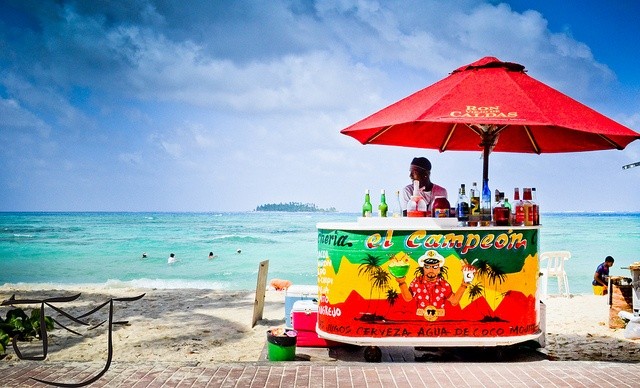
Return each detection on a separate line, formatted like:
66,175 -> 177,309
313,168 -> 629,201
340,56 -> 640,190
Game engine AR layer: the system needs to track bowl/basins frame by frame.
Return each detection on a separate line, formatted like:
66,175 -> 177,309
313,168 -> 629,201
389,264 -> 411,278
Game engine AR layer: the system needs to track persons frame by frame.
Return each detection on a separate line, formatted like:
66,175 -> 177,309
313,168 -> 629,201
395,249 -> 471,322
236,249 -> 242,255
142,252 -> 148,259
168,252 -> 176,263
592,256 -> 614,289
403,157 -> 448,217
208,251 -> 215,258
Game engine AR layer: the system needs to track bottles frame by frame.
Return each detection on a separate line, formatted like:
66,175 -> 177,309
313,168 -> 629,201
458,184 -> 470,226
495,192 -> 508,225
512,187 -> 522,225
406,195 -> 428,218
392,191 -> 402,218
378,189 -> 388,217
431,195 -> 450,218
468,188 -> 476,227
480,179 -> 491,226
527,188 -> 535,208
363,189 -> 372,217
492,189 -> 501,223
532,187 -> 539,225
503,198 -> 512,225
456,188 -> 464,227
523,188 -> 534,226
467,182 -> 480,227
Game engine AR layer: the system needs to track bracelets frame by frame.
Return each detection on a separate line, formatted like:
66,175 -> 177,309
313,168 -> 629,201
399,281 -> 406,286
460,283 -> 468,288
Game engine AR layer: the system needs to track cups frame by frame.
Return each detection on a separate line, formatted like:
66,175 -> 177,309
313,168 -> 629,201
462,264 -> 475,283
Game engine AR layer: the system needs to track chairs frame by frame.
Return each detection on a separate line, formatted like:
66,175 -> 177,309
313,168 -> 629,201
541,249 -> 571,298
